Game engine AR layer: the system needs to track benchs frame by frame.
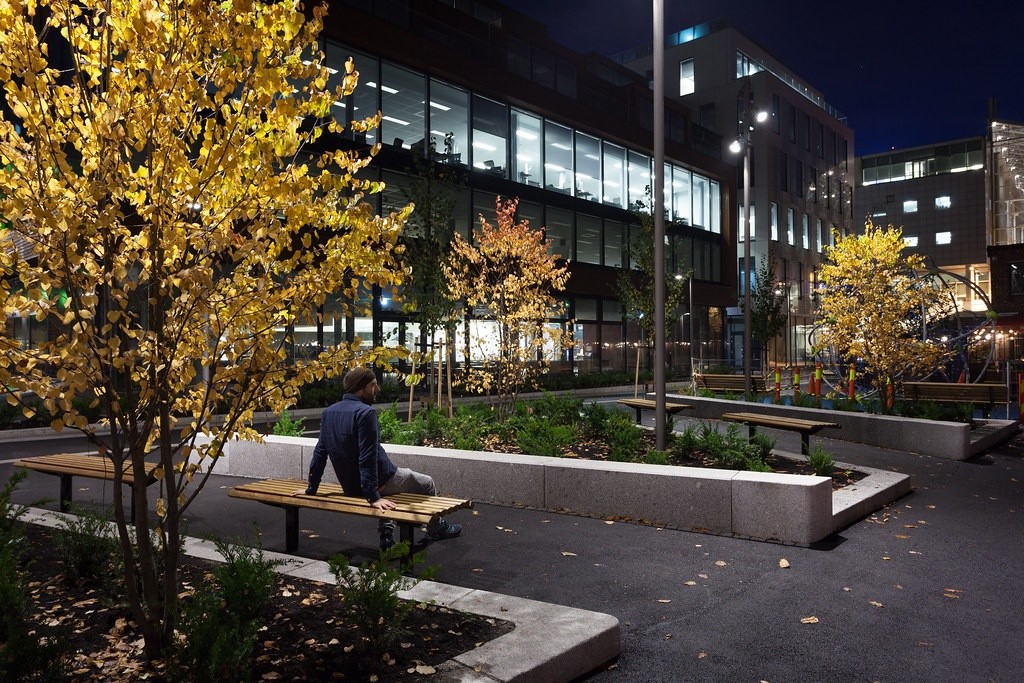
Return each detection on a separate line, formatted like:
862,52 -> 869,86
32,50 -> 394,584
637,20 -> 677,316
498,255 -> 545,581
722,412 -> 842,455
902,381 -> 1012,418
229,478 -> 475,574
616,398 -> 696,430
694,373 -> 773,396
13,453 -> 180,528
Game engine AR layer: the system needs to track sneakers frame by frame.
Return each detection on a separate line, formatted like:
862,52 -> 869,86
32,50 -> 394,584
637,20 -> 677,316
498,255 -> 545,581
380,532 -> 394,551
426,517 -> 462,541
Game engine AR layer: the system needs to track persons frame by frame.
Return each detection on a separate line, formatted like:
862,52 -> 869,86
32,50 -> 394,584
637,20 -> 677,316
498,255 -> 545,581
291,367 -> 462,551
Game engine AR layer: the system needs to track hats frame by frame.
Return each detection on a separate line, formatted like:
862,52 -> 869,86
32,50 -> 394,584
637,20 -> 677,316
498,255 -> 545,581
343,366 -> 374,394
